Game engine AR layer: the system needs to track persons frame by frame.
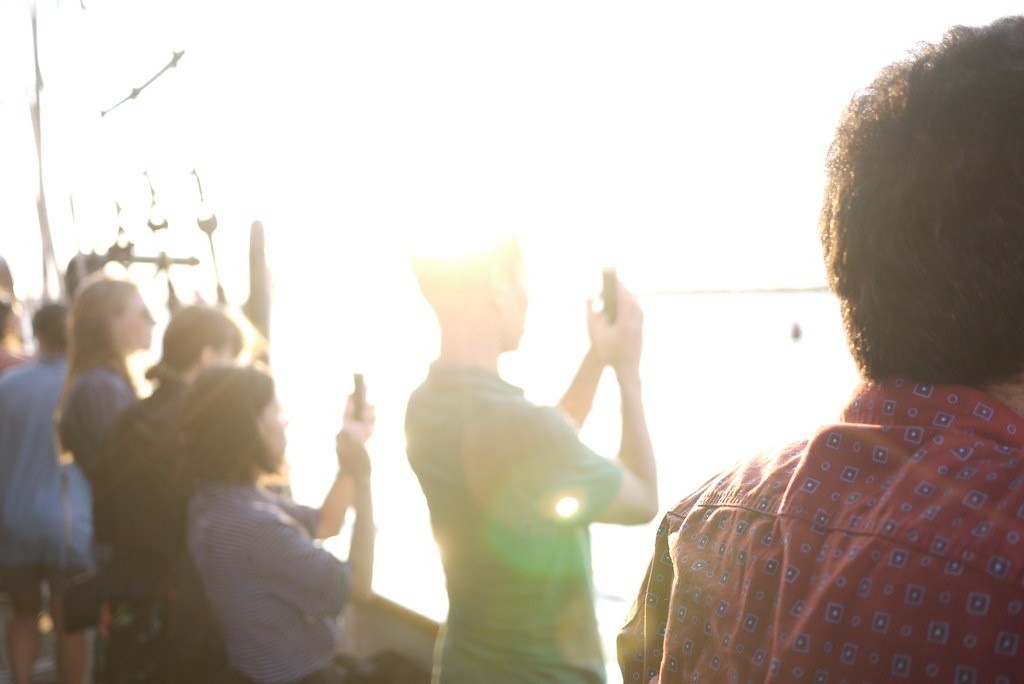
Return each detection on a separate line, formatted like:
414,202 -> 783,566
401,208 -> 661,684
0,244 -> 383,683
615,14 -> 1024,684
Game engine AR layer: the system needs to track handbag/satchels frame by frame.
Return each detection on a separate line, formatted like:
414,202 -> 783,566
63,572 -> 100,634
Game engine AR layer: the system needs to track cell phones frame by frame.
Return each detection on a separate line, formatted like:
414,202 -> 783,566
602,269 -> 618,326
353,372 -> 365,421
